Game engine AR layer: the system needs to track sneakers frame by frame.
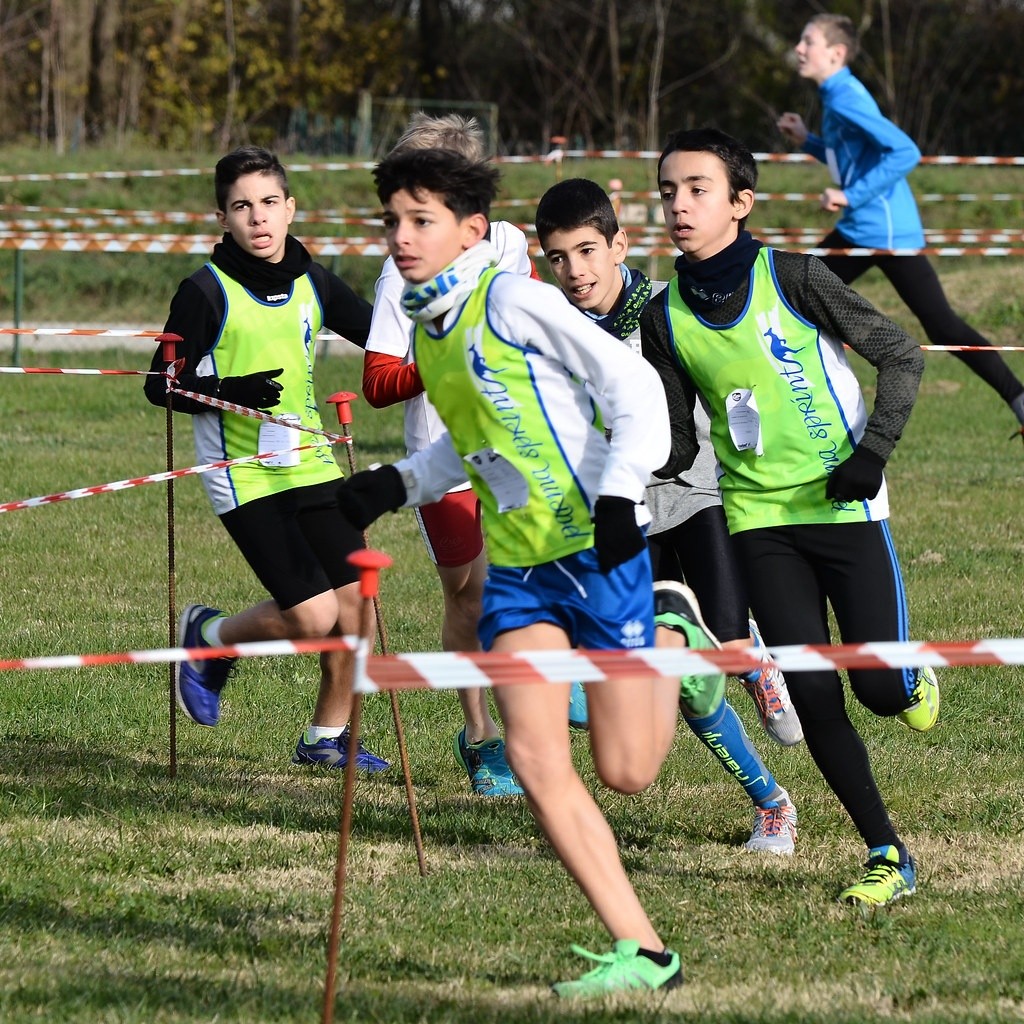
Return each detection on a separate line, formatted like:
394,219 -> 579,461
837,841 -> 917,908
734,619 -> 804,748
453,721 -> 526,798
742,785 -> 798,855
896,666 -> 941,733
173,604 -> 242,728
652,580 -> 728,721
550,938 -> 685,1006
290,720 -> 391,774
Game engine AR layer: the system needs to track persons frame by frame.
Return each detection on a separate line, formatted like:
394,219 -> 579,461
143,147 -> 395,774
639,129 -> 940,910
362,112 -> 726,1002
776,13 -> 1023,443
536,179 -> 805,854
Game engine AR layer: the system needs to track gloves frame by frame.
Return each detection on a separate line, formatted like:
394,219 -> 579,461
337,465 -> 408,533
217,369 -> 285,415
591,495 -> 648,577
824,444 -> 887,504
